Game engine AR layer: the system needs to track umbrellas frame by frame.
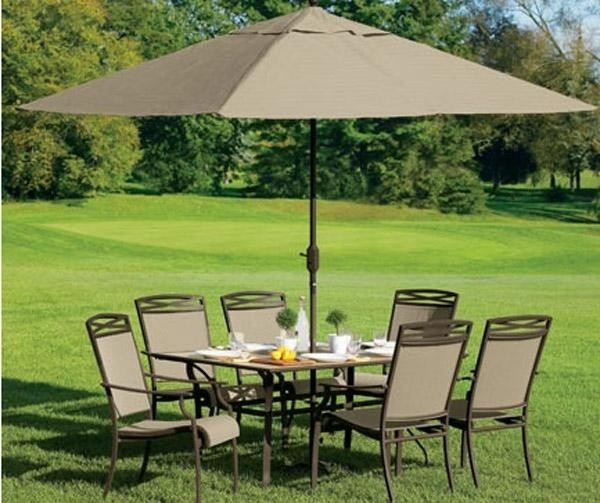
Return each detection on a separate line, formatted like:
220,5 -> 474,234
16,5 -> 599,422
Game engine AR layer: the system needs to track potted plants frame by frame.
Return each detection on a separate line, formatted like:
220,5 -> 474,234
275,308 -> 299,351
325,309 -> 349,353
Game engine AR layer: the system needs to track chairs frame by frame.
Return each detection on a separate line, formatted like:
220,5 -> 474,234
86,312 -> 241,503
220,291 -> 347,454
340,288 -> 460,467
432,314 -> 553,487
309,319 -> 473,503
134,294 -> 262,457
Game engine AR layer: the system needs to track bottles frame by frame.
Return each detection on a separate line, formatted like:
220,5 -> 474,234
296,295 -> 310,351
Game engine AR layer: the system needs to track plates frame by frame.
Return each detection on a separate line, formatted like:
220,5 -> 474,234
362,340 -> 396,347
197,348 -> 244,360
242,342 -> 280,353
300,352 -> 350,364
364,347 -> 394,357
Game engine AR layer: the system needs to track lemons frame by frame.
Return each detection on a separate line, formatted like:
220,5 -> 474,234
271,347 -> 296,360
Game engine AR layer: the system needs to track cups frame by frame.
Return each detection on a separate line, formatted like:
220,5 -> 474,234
347,334 -> 361,357
226,331 -> 244,352
371,327 -> 388,348
